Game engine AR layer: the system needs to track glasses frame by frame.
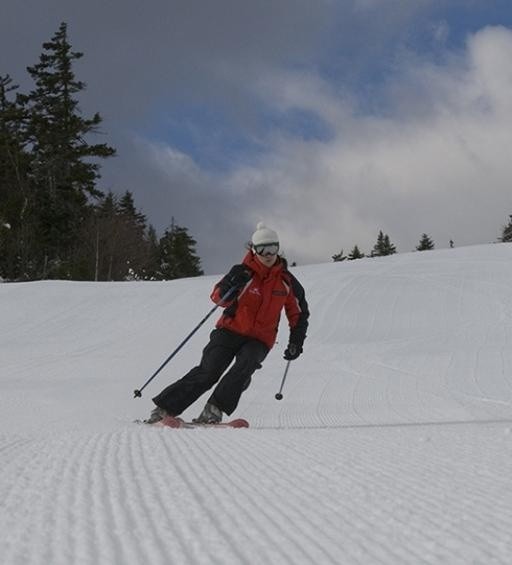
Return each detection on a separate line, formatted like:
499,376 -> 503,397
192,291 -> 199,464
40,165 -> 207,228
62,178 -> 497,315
251,242 -> 280,257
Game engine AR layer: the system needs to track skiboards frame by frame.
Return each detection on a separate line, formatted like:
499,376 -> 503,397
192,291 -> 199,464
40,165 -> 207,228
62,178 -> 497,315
134,416 -> 251,427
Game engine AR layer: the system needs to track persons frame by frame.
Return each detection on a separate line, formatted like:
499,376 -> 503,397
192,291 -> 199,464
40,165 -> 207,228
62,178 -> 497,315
145,221 -> 310,424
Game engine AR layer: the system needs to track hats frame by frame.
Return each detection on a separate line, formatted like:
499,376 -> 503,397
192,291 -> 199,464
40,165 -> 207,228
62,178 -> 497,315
251,223 -> 280,255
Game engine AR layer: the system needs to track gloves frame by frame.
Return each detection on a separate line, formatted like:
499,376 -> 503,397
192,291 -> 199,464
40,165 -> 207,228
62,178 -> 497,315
229,270 -> 249,290
284,342 -> 302,361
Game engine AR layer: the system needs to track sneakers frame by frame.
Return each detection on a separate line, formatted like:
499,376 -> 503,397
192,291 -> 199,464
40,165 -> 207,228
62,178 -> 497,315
150,406 -> 172,422
200,403 -> 223,423
